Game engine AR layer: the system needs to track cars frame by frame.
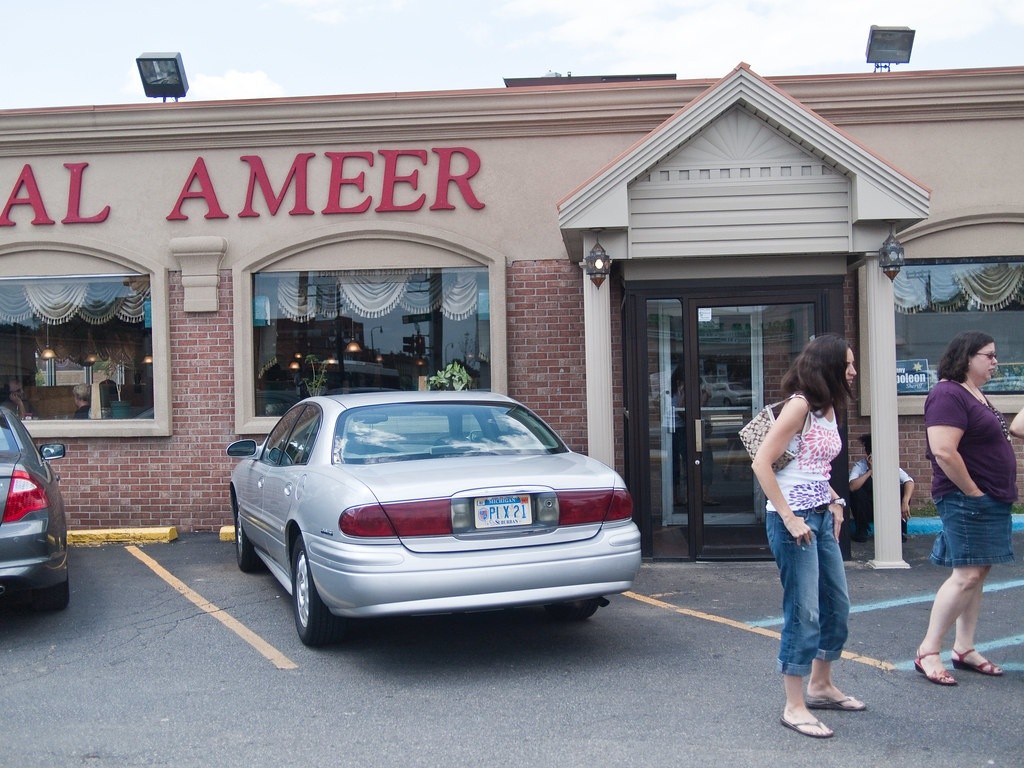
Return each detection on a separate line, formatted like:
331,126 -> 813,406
704,381 -> 752,407
0,404 -> 70,616
225,389 -> 643,645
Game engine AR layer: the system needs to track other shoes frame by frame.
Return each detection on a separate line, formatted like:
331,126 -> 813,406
703,498 -> 720,505
854,527 -> 868,542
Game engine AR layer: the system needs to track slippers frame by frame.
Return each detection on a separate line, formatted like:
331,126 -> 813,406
780,714 -> 833,737
807,696 -> 867,711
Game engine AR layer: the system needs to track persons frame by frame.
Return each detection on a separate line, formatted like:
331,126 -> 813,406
1008,408 -> 1024,442
69,383 -> 92,420
651,364 -> 715,505
848,438 -> 915,543
751,334 -> 867,738
2,380 -> 28,420
913,329 -> 1019,685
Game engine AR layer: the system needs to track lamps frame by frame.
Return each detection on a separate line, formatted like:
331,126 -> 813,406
878,219 -> 904,282
865,25 -> 916,74
585,228 -> 610,290
136,52 -> 189,104
344,313 -> 362,353
39,321 -> 57,359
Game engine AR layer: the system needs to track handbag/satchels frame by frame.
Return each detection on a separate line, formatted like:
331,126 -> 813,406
738,394 -> 810,473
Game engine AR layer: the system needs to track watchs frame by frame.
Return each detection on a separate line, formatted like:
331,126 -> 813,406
832,498 -> 846,507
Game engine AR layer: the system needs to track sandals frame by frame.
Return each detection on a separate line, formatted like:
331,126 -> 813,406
952,649 -> 1002,675
914,648 -> 958,684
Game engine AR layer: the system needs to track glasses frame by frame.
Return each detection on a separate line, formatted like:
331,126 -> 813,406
976,352 -> 998,359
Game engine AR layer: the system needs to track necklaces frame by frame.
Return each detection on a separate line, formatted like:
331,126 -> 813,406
964,380 -> 1012,442
